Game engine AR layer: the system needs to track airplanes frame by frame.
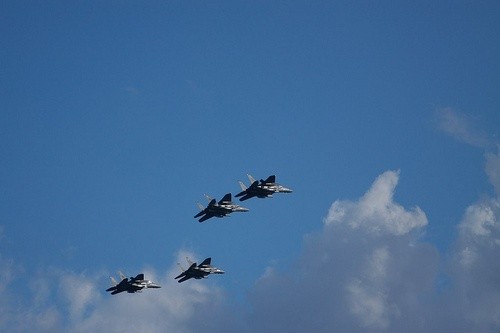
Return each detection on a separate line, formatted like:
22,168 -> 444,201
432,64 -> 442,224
104,271 -> 162,295
192,192 -> 251,223
234,173 -> 293,202
174,254 -> 225,284
